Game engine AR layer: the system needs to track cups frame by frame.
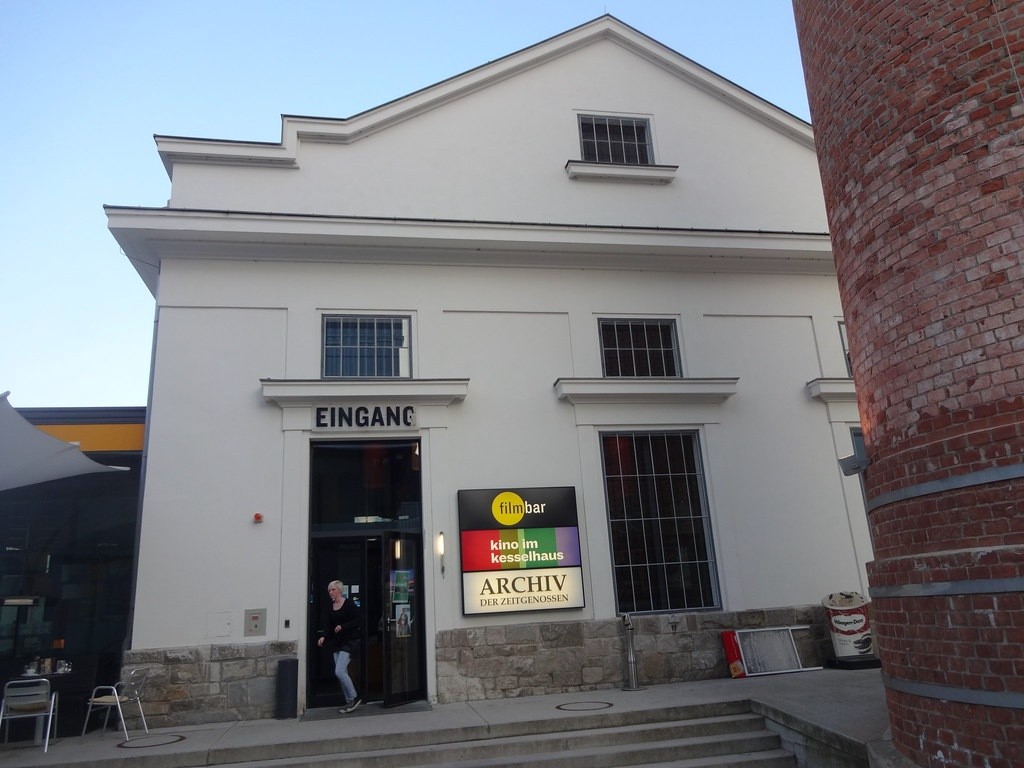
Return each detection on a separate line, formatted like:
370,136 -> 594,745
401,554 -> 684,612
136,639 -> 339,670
57,660 -> 66,672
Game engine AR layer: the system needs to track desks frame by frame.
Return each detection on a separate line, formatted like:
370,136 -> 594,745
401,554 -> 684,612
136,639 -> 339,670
16,672 -> 78,747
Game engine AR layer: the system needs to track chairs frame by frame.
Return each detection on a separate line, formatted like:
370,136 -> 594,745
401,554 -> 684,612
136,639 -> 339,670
0,679 -> 58,753
82,667 -> 150,742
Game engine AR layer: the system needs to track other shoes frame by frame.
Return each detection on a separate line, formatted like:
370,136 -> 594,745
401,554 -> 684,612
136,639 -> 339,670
346,696 -> 362,712
339,704 -> 346,713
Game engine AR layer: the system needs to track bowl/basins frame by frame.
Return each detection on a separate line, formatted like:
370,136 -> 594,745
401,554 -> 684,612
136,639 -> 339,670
26,669 -> 35,674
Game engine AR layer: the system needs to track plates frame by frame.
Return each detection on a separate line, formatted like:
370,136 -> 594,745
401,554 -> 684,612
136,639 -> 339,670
21,674 -> 38,677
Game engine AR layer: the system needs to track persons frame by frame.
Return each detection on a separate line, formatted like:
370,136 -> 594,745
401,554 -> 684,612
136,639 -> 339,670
318,580 -> 362,713
